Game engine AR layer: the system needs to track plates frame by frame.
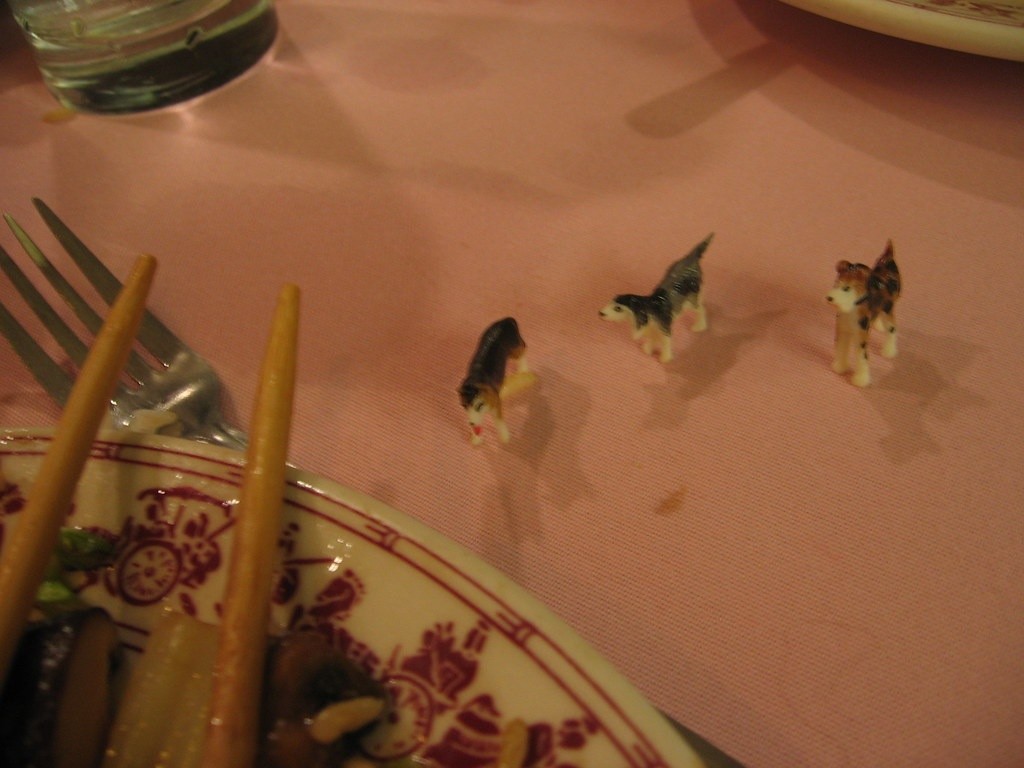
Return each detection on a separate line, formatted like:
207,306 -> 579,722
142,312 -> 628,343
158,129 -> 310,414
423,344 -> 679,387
1,428 -> 708,768
783,1 -> 1024,64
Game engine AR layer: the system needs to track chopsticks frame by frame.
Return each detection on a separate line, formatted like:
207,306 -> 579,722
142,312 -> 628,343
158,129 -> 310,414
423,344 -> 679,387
1,254 -> 301,768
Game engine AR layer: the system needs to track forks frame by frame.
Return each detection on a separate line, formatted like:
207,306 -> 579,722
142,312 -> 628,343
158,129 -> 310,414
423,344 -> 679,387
0,198 -> 747,768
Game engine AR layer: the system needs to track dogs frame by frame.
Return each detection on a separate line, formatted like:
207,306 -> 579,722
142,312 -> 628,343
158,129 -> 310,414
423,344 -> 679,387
454,316 -> 527,446
824,237 -> 902,386
597,230 -> 717,364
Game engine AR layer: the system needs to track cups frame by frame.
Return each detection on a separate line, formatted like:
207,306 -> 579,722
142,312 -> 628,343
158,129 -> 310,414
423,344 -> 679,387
10,0 -> 282,115
